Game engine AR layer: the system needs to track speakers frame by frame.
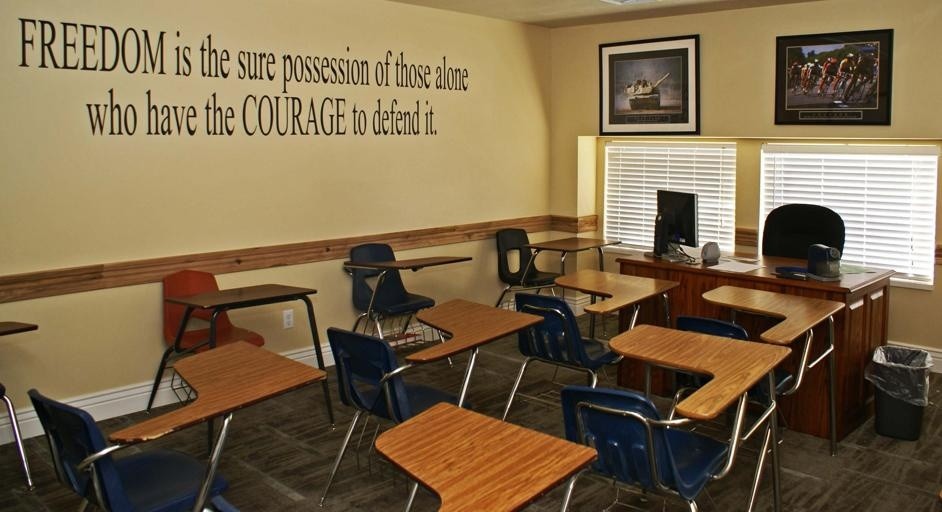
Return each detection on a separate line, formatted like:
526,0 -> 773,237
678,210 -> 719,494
701,242 -> 721,263
666,243 -> 680,250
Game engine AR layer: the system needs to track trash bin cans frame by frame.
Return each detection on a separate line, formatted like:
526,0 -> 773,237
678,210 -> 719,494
871,345 -> 931,441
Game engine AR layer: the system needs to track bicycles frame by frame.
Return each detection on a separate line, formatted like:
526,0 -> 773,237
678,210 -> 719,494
791,68 -> 871,105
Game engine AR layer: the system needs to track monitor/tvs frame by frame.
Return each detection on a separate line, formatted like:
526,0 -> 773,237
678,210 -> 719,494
644,190 -> 699,263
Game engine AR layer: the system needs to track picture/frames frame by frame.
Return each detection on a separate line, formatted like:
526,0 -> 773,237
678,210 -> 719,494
597,32 -> 701,137
773,29 -> 892,127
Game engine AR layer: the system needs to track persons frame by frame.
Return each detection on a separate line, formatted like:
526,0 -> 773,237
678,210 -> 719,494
788,50 -> 878,106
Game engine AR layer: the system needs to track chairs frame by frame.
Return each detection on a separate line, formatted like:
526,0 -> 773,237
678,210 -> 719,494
495,228 -> 561,310
762,203 -> 846,260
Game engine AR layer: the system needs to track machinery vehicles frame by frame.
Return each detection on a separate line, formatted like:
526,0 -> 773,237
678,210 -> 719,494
622,71 -> 672,109
